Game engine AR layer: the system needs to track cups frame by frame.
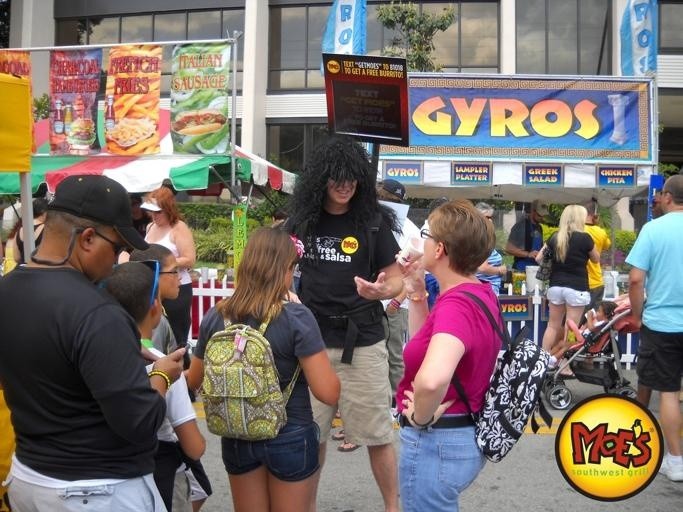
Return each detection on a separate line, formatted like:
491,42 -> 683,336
200,266 -> 208,284
397,235 -> 423,268
216,265 -> 225,282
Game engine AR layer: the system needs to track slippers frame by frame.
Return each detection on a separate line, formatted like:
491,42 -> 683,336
332,428 -> 360,452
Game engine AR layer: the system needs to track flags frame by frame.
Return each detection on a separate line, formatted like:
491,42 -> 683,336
319,0 -> 367,78
621,0 -> 661,82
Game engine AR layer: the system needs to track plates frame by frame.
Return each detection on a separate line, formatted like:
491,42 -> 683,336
110,118 -> 153,148
170,112 -> 227,137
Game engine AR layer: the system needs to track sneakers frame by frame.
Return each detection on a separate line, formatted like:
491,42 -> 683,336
654,452 -> 683,481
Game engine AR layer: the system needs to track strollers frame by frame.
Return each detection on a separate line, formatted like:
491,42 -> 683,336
536,305 -> 642,412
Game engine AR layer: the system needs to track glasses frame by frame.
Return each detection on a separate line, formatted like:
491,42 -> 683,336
420,228 -> 438,242
89,226 -> 127,259
650,190 -> 673,208
485,216 -> 494,219
97,259 -> 180,304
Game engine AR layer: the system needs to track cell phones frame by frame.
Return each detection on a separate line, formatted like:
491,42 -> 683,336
170,342 -> 189,354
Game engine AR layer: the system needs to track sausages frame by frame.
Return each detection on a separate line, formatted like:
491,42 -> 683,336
178,122 -> 222,134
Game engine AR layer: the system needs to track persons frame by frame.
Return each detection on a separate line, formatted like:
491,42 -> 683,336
0,185 -> 303,404
576,199 -> 610,321
625,172 -> 682,480
634,188 -> 669,408
475,202 -> 506,299
377,176 -> 423,417
533,205 -> 599,361
330,423 -> 361,452
0,172 -> 170,511
106,262 -> 207,510
286,136 -> 406,510
394,200 -> 503,510
185,229 -> 340,511
506,199 -> 550,273
418,195 -> 450,314
129,241 -> 213,511
551,302 -> 616,360
0,385 -> 16,511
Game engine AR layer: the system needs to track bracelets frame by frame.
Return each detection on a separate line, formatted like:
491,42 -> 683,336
498,266 -> 501,274
147,370 -> 169,390
411,410 -> 434,426
407,290 -> 430,302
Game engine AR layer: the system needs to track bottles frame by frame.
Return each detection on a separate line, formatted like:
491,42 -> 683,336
103,95 -> 116,132
602,271 -> 615,298
52,100 -> 73,136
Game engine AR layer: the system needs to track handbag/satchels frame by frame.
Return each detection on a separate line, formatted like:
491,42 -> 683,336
535,246 -> 553,282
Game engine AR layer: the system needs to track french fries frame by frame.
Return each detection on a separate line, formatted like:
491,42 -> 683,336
106,45 -> 159,155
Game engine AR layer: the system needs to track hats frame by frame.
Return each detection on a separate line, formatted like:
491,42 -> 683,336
139,197 -> 165,213
378,178 -> 411,205
532,199 -> 550,217
47,174 -> 151,253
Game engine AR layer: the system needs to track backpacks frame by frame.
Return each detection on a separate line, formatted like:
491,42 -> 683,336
201,299 -> 302,442
450,291 -> 550,465
